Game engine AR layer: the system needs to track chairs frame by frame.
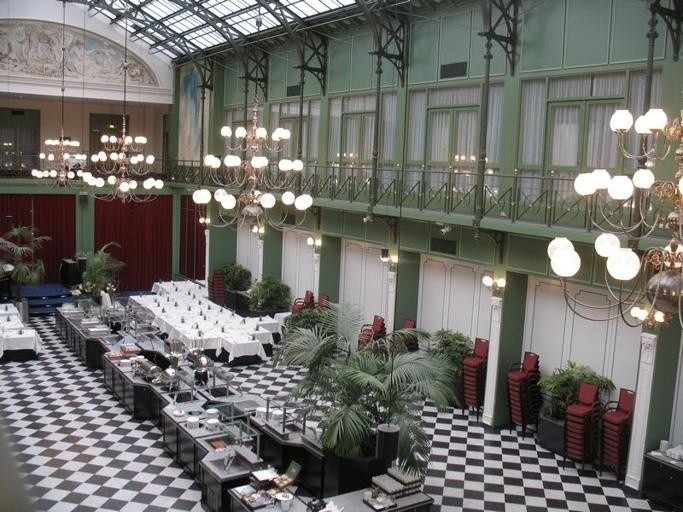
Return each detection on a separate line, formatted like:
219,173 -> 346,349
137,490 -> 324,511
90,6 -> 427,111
292,291 -> 329,316
506,351 -> 542,438
597,388 -> 636,486
563,382 -> 604,474
462,335 -> 488,423
209,268 -> 226,305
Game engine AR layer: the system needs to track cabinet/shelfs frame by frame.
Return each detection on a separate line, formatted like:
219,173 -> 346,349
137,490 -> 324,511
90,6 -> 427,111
641,448 -> 682,512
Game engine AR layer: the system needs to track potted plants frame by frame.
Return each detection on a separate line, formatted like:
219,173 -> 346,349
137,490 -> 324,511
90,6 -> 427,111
224,265 -> 250,310
536,359 -> 617,459
267,297 -> 461,481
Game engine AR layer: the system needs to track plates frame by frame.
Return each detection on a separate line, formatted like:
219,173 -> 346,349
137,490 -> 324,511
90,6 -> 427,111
255,407 -> 273,419
187,418 -> 199,428
130,354 -> 145,364
172,408 -> 185,416
206,408 -> 219,419
272,411 -> 283,421
207,420 -> 219,430
276,492 -> 293,501
120,360 -> 132,367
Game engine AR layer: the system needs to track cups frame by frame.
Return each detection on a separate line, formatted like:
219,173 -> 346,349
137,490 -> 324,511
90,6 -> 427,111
281,499 -> 290,511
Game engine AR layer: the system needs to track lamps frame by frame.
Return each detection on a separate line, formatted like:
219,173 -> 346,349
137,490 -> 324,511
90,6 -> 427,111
380,248 -> 398,272
193,0 -> 313,242
628,306 -> 670,336
199,217 -> 210,237
547,0 -> 683,328
251,225 -> 264,248
481,273 -> 506,308
306,237 -> 321,263
31,0 -> 94,192
88,1 -> 164,206
